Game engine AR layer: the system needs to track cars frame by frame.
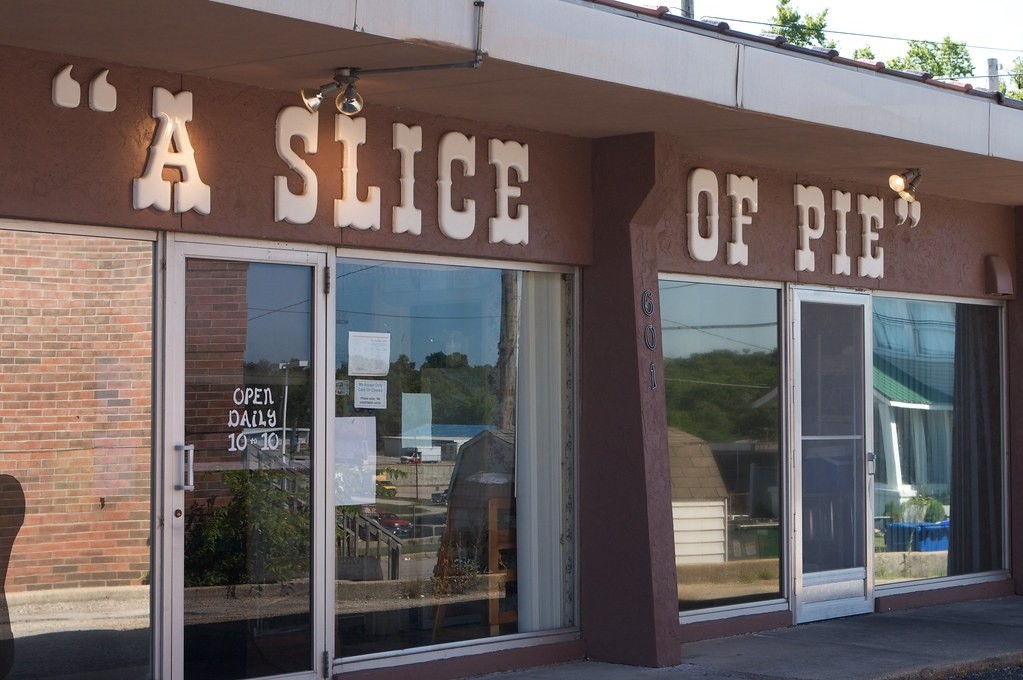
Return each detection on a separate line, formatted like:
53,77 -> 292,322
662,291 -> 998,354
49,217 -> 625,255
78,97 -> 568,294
378,513 -> 413,537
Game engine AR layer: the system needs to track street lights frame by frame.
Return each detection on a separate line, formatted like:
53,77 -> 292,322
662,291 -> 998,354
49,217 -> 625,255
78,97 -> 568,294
272,360 -> 308,507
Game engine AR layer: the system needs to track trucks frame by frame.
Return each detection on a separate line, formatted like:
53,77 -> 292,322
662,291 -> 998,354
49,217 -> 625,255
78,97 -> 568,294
401,447 -> 442,464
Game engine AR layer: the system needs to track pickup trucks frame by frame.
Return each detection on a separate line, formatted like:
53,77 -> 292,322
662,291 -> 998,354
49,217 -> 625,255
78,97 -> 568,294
432,489 -> 449,505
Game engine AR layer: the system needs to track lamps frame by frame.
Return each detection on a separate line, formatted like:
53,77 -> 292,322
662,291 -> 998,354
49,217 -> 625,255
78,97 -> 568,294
889,168 -> 922,202
300,77 -> 364,116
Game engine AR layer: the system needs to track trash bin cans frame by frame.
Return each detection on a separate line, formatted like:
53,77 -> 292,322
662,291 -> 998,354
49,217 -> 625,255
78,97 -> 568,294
884,522 -> 941,552
936,520 -> 949,551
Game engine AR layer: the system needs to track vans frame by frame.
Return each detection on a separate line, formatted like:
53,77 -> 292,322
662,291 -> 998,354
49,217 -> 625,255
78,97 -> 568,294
376,480 -> 397,496
